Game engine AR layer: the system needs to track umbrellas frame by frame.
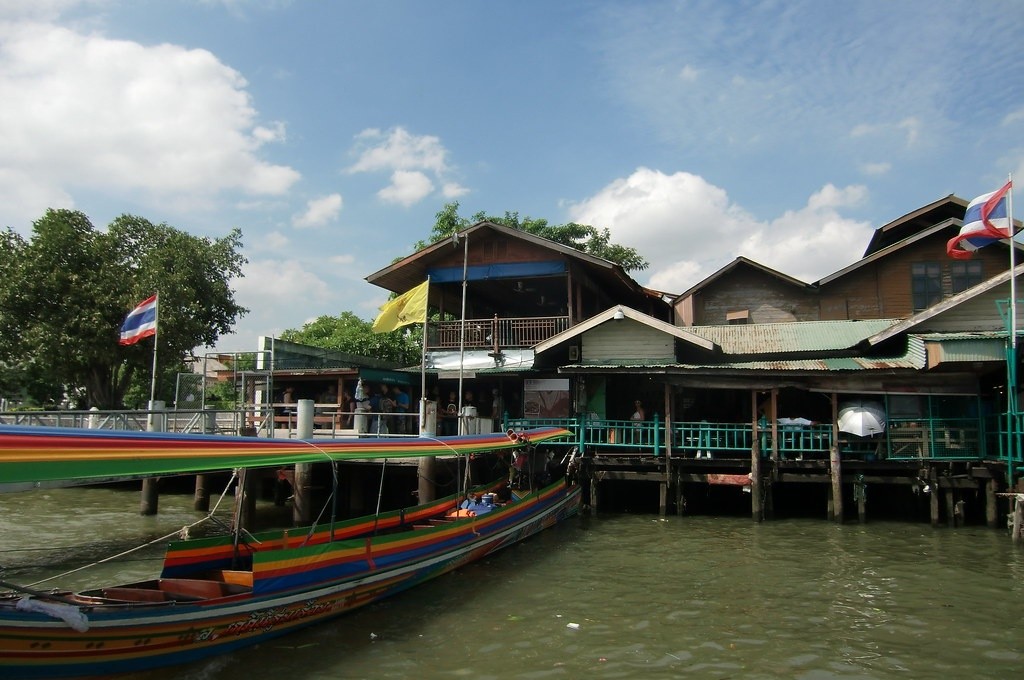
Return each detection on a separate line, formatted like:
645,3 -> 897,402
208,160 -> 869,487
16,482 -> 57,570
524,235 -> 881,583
837,400 -> 886,437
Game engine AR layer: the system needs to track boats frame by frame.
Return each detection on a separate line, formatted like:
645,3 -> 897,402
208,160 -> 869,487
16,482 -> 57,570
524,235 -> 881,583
0,424 -> 585,680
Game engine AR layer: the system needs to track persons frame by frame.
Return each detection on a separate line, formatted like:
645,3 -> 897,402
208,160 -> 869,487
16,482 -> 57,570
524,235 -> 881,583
413,388 -> 429,434
630,399 -> 644,443
44,393 -> 54,405
315,383 -> 338,429
547,461 -> 565,483
274,385 -> 296,423
777,413 -> 821,438
185,390 -> 194,402
507,450 -> 526,489
344,383 -> 410,434
444,388 -> 505,435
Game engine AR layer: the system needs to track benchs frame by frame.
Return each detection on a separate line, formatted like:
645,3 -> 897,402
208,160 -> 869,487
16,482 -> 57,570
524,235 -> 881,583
407,515 -> 467,530
101,569 -> 254,603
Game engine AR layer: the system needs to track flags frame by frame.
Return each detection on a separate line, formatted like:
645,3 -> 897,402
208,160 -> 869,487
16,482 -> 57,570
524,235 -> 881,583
959,190 -> 1010,251
371,280 -> 429,334
119,296 -> 156,345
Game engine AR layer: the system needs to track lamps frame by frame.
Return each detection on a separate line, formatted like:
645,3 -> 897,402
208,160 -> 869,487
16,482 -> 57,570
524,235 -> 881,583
614,310 -> 624,319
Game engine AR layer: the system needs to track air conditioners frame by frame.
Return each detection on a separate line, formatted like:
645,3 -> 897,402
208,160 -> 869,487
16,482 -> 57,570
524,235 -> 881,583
569,346 -> 579,361
